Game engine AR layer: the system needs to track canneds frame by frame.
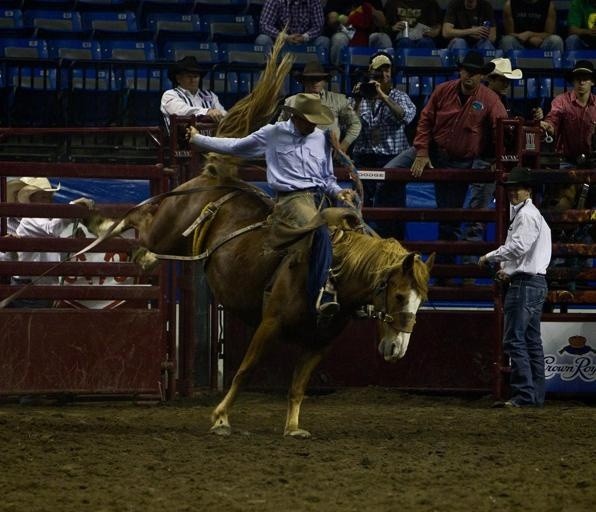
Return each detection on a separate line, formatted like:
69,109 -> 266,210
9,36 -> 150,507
399,21 -> 408,39
480,20 -> 491,40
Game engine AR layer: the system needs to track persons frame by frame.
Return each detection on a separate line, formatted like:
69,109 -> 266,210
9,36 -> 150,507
8,177 -> 93,308
159,55 -> 228,192
184,93 -> 376,320
477,166 -> 553,410
0,177 -> 34,262
256,0 -> 596,290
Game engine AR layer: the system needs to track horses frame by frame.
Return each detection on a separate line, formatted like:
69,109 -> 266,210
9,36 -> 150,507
81,19 -> 436,439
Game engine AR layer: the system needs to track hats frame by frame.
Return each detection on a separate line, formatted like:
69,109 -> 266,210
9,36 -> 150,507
167,56 -> 208,83
293,60 -> 338,81
490,57 -> 523,79
279,93 -> 335,125
6,177 -> 61,204
500,167 -> 534,188
568,60 -> 596,78
456,50 -> 495,75
368,55 -> 392,71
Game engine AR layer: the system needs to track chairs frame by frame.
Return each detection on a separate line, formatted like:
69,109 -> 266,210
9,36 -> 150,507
0,2 -> 596,108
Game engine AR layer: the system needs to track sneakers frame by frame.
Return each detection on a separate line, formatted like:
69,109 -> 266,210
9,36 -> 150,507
312,302 -> 340,336
